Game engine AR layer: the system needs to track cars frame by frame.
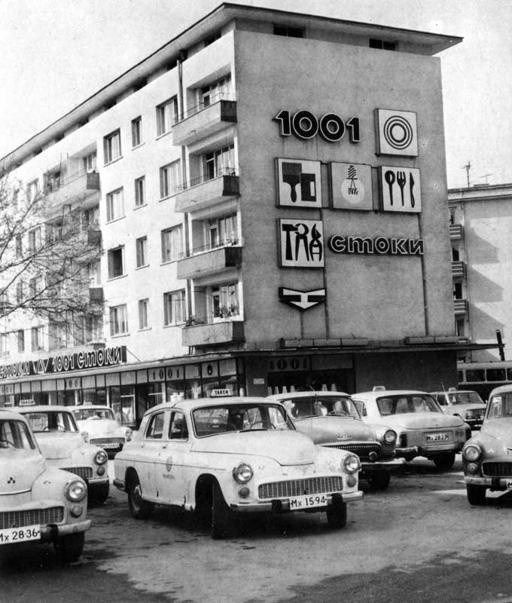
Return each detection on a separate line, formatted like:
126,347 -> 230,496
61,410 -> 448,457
461,384 -> 512,507
64,402 -> 135,460
0,399 -> 110,509
0,410 -> 91,563
429,387 -> 488,431
113,389 -> 364,541
265,390 -> 407,491
351,386 -> 471,473
